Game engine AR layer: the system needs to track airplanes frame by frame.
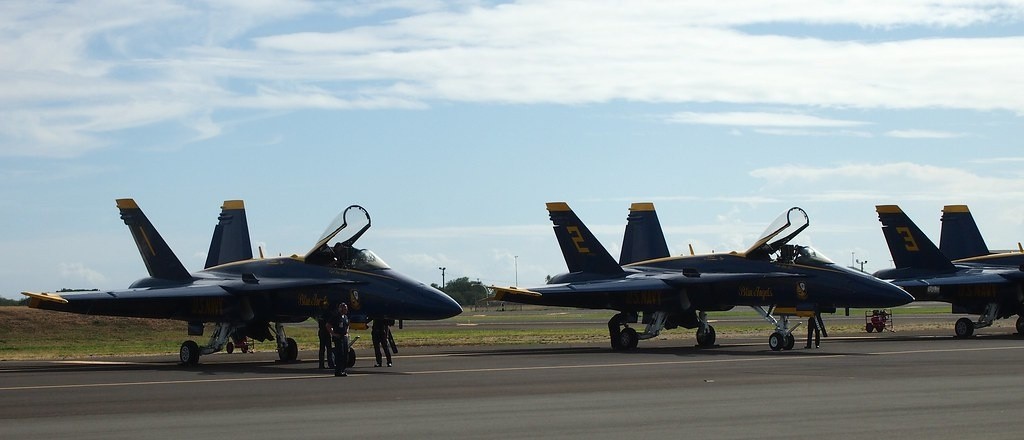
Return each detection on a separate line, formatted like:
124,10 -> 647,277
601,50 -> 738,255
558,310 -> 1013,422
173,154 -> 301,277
486,200 -> 918,351
866,203 -> 1024,340
20,196 -> 463,365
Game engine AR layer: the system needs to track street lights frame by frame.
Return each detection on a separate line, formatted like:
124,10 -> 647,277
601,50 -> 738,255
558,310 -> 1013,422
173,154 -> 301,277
514,255 -> 519,287
470,283 -> 489,307
438,266 -> 447,291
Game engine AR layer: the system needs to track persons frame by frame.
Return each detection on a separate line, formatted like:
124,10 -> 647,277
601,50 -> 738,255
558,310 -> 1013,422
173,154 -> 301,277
318,311 -> 336,369
608,311 -> 629,351
803,317 -> 820,349
371,319 -> 395,367
325,303 -> 350,377
334,242 -> 344,251
795,245 -> 810,258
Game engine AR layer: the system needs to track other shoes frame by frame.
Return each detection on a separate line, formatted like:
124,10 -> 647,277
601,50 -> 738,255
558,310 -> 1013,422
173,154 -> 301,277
386,363 -> 392,367
374,363 -> 382,367
335,372 -> 347,377
